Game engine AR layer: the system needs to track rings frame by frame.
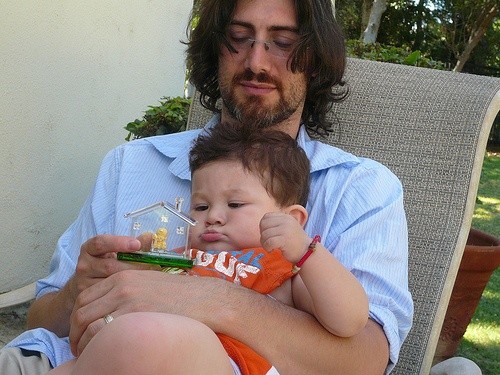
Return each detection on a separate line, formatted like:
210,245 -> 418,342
104,314 -> 113,325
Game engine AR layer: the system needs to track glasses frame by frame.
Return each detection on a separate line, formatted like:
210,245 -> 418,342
218,32 -> 303,57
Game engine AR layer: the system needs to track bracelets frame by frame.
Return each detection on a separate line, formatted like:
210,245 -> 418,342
292,234 -> 321,275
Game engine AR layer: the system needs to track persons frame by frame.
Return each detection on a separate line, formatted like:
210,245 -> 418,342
45,121 -> 371,375
1,0 -> 413,375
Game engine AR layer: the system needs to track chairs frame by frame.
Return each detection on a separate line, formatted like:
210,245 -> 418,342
186,56 -> 500,375
